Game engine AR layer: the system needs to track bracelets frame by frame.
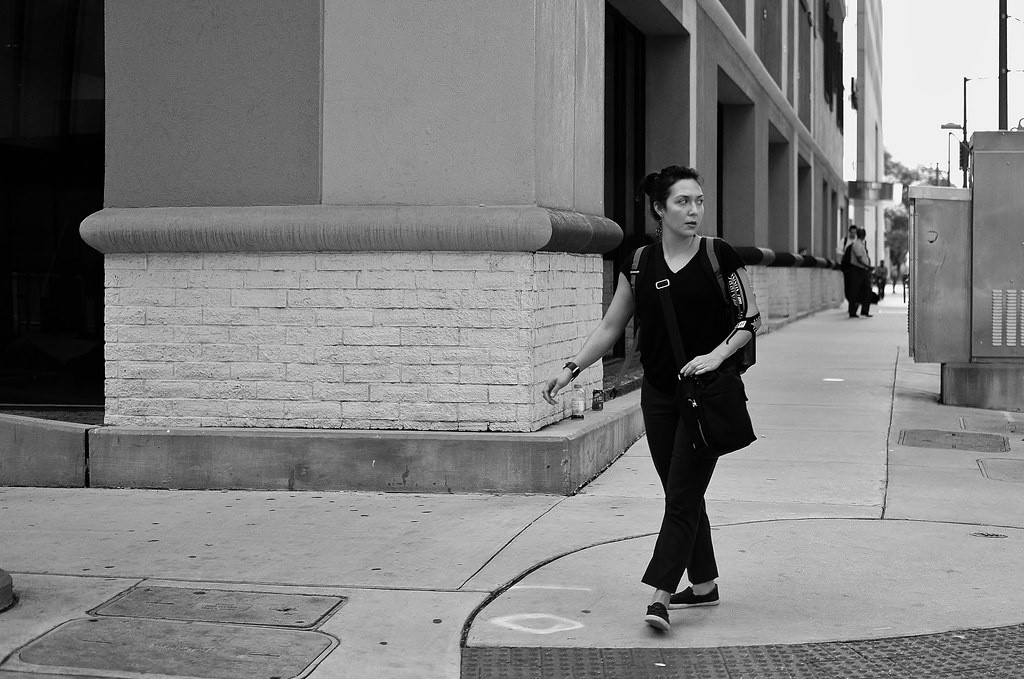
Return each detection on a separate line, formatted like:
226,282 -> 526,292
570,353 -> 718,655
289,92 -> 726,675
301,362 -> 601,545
563,362 -> 580,382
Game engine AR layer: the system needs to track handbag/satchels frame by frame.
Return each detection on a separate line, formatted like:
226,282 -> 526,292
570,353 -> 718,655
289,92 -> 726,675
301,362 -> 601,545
683,372 -> 757,459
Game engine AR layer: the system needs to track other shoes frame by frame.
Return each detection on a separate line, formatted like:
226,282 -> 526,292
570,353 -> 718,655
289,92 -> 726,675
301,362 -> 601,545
667,583 -> 719,608
849,314 -> 859,317
645,602 -> 670,631
861,313 -> 872,317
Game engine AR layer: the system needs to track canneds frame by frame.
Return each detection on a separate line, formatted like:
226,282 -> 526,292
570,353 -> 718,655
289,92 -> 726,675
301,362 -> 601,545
592,389 -> 604,411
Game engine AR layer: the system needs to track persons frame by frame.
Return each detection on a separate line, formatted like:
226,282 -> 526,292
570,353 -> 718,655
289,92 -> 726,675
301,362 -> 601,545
874,260 -> 887,299
836,225 -> 874,318
890,262 -> 897,293
900,261 -> 909,285
541,166 -> 761,631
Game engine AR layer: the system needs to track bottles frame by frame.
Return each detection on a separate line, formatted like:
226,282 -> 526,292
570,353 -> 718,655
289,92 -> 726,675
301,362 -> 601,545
572,385 -> 585,416
